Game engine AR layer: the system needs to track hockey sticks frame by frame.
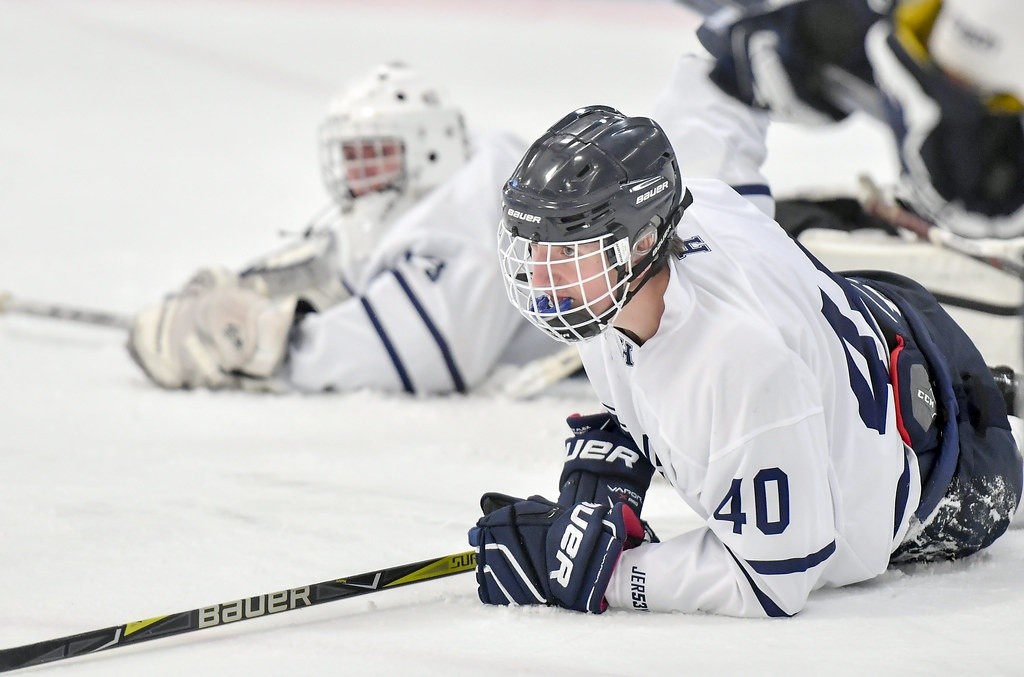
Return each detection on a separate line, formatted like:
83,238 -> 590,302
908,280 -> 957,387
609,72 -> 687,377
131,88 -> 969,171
0,293 -> 136,330
930,289 -> 1024,318
0,545 -> 478,672
890,206 -> 1024,283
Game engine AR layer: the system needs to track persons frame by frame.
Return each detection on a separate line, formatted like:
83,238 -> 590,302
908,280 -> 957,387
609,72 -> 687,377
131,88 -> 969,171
124,0 -> 1024,409
469,106 -> 1024,619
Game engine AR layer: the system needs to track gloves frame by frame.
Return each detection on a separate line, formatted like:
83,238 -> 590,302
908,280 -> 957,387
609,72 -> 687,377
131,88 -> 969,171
126,268 -> 320,395
557,413 -> 656,518
468,493 -> 646,614
236,231 -> 349,309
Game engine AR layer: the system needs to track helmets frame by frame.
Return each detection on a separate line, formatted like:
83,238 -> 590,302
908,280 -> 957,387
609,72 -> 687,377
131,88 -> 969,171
316,61 -> 471,225
495,105 -> 694,346
